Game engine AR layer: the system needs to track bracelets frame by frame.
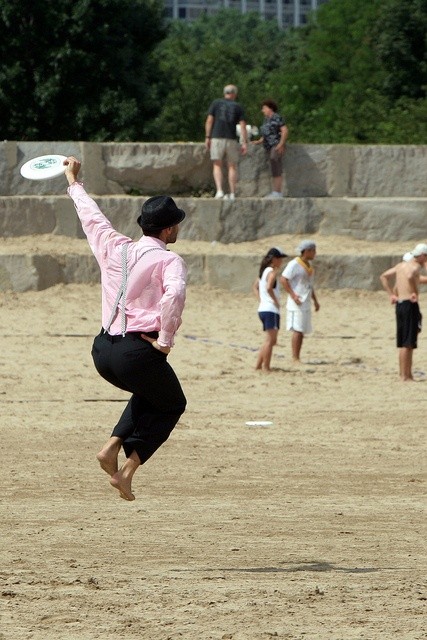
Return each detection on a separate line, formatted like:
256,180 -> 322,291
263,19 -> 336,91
204,135 -> 211,139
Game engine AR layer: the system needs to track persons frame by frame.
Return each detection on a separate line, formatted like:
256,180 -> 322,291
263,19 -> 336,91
252,247 -> 289,370
249,100 -> 288,199
203,84 -> 248,200
280,240 -> 320,361
389,252 -> 427,377
380,244 -> 427,380
61,155 -> 187,501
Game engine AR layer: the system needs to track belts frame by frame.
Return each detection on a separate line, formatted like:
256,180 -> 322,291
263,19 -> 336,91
102,330 -> 121,343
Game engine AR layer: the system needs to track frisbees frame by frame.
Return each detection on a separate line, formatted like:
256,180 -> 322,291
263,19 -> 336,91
20,155 -> 69,180
245,421 -> 272,426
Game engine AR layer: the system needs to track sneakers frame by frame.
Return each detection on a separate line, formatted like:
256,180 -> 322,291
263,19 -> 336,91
224,192 -> 235,199
263,191 -> 283,197
214,191 -> 224,200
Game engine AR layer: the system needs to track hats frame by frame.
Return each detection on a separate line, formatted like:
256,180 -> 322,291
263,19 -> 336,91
411,244 -> 426,256
268,247 -> 287,258
295,240 -> 315,252
136,196 -> 185,230
